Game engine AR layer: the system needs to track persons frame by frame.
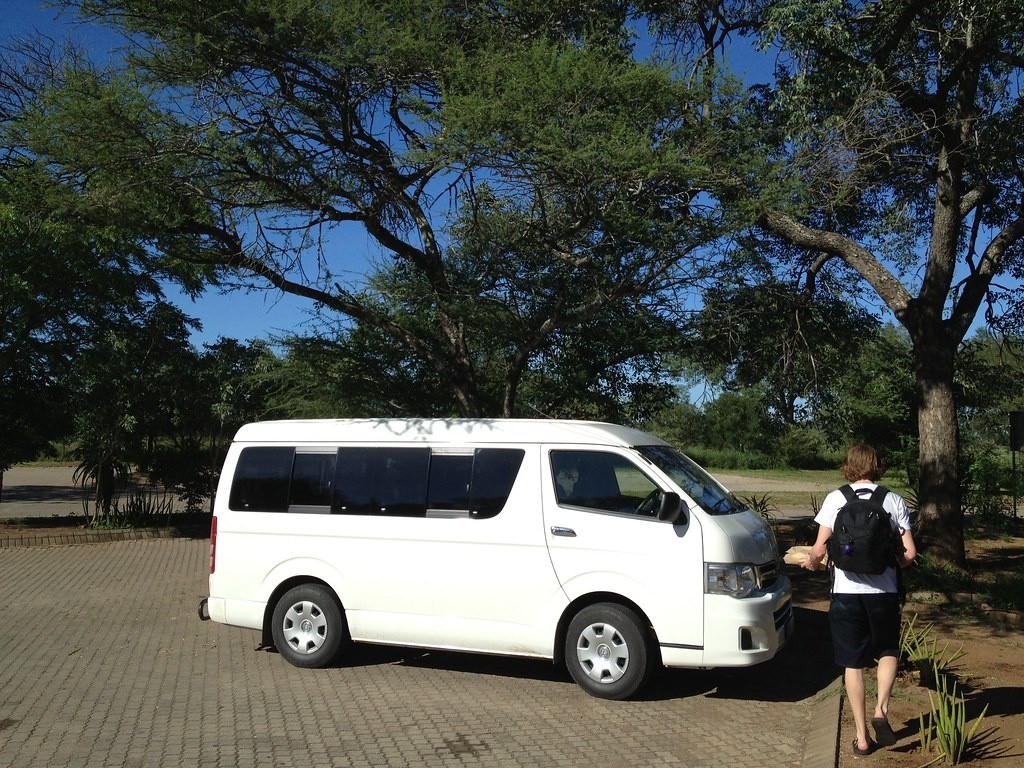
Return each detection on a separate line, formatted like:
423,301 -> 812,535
801,446 -> 915,757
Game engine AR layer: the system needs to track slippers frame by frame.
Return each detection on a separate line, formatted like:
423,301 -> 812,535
852,737 -> 872,755
871,718 -> 896,744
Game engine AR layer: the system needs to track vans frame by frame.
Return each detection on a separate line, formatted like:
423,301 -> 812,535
198,417 -> 795,700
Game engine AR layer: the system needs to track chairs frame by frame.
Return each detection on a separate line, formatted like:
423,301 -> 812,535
578,454 -> 627,511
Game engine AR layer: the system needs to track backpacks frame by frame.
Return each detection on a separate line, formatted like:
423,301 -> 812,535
823,484 -> 907,606
